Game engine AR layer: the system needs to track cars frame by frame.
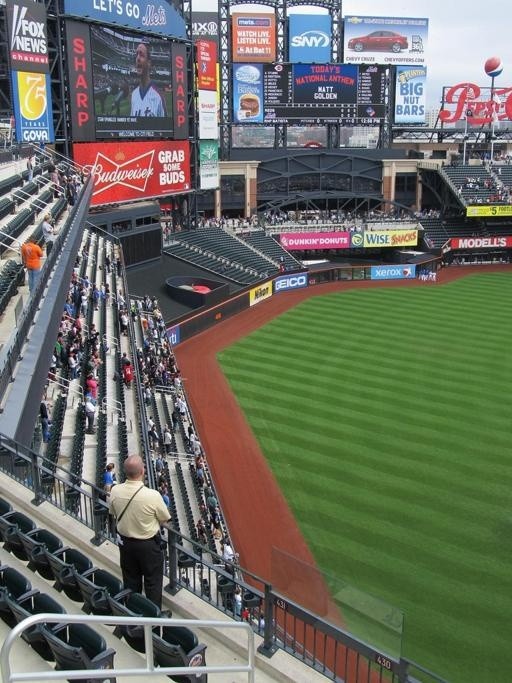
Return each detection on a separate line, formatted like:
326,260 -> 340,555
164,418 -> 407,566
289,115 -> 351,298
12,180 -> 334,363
348,29 -> 408,53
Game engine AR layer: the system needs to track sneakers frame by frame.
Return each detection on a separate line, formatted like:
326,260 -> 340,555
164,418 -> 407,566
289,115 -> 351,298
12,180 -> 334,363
161,609 -> 172,618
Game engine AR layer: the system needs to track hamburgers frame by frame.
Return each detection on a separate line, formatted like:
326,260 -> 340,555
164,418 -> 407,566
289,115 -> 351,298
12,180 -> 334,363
240,94 -> 259,117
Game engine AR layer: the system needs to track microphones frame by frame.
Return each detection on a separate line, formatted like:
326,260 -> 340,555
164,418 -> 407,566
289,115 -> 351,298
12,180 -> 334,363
94,83 -> 119,100
111,84 -> 129,109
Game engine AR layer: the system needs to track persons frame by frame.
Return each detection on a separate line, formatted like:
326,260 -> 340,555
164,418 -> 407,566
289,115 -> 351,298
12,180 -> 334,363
26,156 -> 440,608
453,150 -> 511,206
93,43 -> 169,119
